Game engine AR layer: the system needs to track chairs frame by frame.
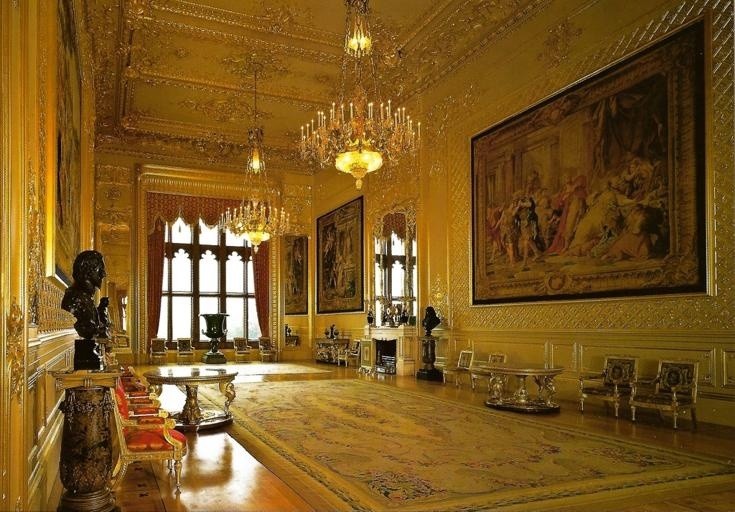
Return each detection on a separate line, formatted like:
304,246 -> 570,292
176,337 -> 196,363
149,338 -> 168,363
232,337 -> 252,363
103,357 -> 190,496
443,350 -> 475,389
577,351 -> 640,421
337,339 -> 362,368
472,353 -> 509,391
629,356 -> 703,429
259,336 -> 275,363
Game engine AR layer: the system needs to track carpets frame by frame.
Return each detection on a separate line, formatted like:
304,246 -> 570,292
155,357 -> 332,375
177,378 -> 734,511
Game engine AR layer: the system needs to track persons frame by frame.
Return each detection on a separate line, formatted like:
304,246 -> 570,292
422,307 -> 440,330
60,250 -> 109,372
322,222 -> 337,291
482,153 -> 655,270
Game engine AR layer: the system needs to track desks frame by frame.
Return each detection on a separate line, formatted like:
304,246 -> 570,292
477,360 -> 565,416
141,363 -> 239,433
314,337 -> 350,366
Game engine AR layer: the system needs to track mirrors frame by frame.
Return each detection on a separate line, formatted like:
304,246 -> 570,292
365,197 -> 420,327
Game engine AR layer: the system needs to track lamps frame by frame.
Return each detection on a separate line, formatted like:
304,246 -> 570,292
212,63 -> 302,252
297,1 -> 421,194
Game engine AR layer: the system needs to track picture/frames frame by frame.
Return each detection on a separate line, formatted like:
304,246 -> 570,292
316,195 -> 366,315
285,235 -> 308,318
44,0 -> 86,296
468,13 -> 716,310
